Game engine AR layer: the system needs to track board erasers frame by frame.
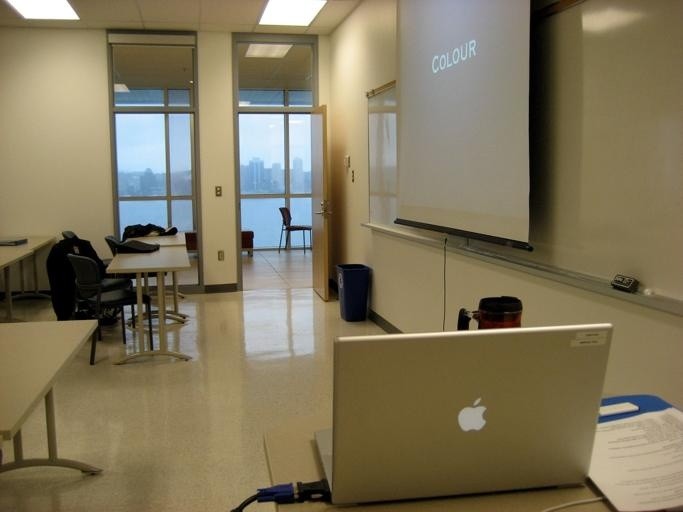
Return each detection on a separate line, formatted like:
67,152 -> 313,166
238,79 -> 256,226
610,274 -> 640,295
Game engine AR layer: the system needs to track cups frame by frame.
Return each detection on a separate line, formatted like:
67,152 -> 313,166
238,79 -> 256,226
453,294 -> 524,331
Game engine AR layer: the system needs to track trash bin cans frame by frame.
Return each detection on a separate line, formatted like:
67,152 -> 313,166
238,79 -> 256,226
336,264 -> 372,322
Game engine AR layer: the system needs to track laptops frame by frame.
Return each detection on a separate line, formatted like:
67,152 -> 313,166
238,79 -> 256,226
315,324 -> 613,506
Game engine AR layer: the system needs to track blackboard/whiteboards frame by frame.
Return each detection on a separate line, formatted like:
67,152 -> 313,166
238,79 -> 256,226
360,0 -> 683,317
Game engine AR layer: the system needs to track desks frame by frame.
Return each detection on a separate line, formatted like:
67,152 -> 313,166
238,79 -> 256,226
0,233 -> 55,302
120,228 -> 186,324
0,245 -> 33,322
104,246 -> 192,365
1,319 -> 101,512
259,430 -> 682,512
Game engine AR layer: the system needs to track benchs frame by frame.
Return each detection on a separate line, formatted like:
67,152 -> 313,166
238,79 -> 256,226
182,228 -> 255,259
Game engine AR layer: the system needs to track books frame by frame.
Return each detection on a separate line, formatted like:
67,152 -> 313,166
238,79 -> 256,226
576,405 -> 682,512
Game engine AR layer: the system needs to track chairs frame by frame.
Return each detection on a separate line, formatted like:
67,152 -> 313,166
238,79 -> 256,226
50,229 -> 137,331
276,206 -> 312,258
46,241 -> 155,363
